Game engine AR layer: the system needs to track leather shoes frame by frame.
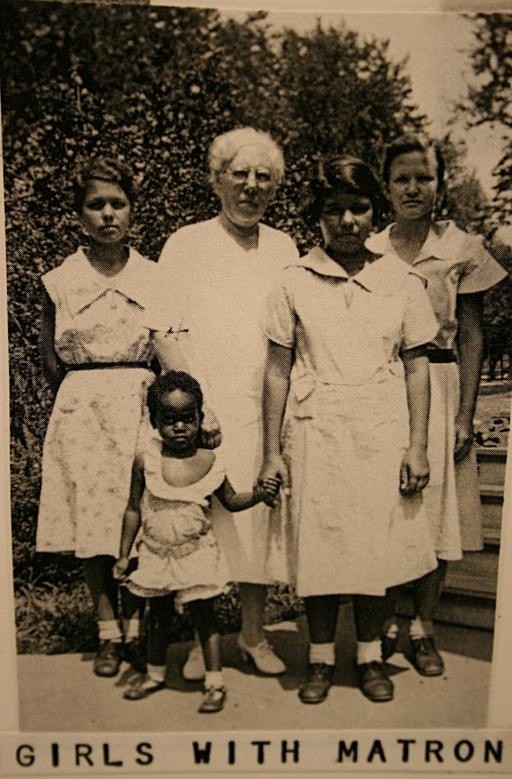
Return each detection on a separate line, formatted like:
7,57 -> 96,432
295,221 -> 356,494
354,660 -> 395,702
198,685 -> 226,711
124,675 -> 168,699
381,631 -> 398,662
94,641 -> 124,676
121,634 -> 150,674
298,661 -> 336,703
410,636 -> 445,677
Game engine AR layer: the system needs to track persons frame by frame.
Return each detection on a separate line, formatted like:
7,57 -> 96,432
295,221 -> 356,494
142,125 -> 307,681
366,132 -> 508,680
253,154 -> 443,704
109,370 -> 282,714
38,154 -> 163,676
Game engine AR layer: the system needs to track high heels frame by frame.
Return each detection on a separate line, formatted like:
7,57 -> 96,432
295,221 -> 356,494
236,631 -> 287,675
182,646 -> 207,680
